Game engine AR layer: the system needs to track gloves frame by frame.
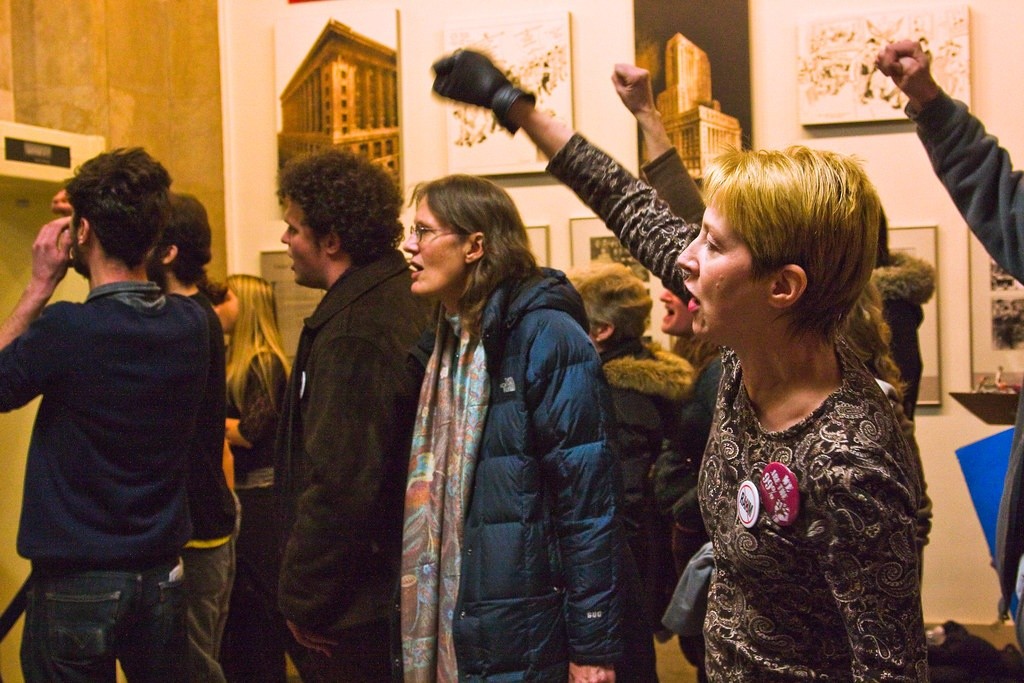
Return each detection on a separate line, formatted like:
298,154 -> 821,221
433,49 -> 536,137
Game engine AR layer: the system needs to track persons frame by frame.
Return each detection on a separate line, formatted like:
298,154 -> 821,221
141,190 -> 238,683
659,290 -> 723,668
402,170 -> 624,683
278,147 -> 442,683
211,272 -> 296,683
430,46 -> 935,682
614,61 -> 936,431
2,147 -> 198,683
565,258 -> 696,683
877,41 -> 1024,651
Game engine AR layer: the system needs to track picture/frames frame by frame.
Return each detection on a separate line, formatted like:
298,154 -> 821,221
793,3 -> 974,131
271,2 -> 405,203
966,227 -> 1024,398
568,215 -> 672,352
883,225 -> 945,409
522,224 -> 552,268
257,250 -> 329,367
442,11 -> 577,179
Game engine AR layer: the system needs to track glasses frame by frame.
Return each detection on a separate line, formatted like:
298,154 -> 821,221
409,224 -> 454,241
